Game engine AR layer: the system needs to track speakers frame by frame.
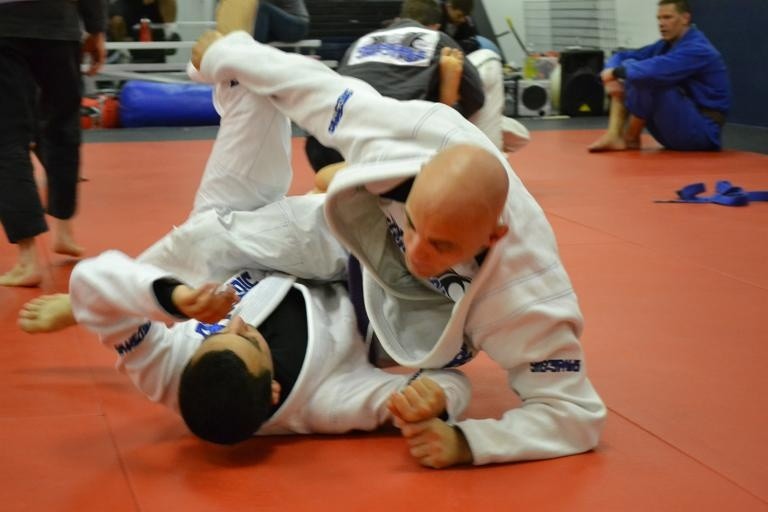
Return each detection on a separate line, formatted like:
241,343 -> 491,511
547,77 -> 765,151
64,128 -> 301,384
559,50 -> 606,116
517,79 -> 552,116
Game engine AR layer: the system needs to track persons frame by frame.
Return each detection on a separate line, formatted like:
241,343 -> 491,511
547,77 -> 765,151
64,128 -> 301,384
16,29 -> 607,471
68,0 -> 472,446
304,1 -> 531,196
106,0 -> 156,42
0,0 -> 104,288
587,0 -> 733,152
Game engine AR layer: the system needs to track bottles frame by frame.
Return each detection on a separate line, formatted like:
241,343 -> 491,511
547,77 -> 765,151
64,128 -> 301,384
138,17 -> 153,41
